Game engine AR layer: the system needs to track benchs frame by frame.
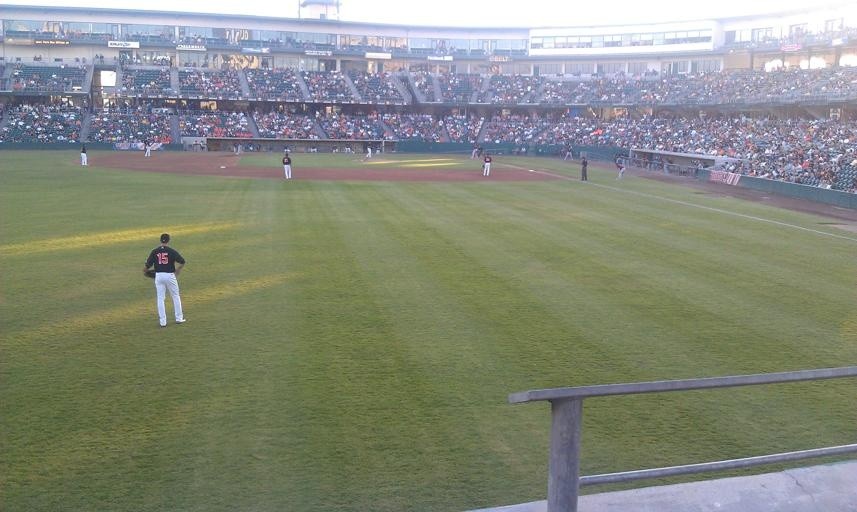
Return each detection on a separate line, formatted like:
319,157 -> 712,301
0,64 -> 857,192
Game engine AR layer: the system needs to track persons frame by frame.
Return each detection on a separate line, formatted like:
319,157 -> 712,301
0,26 -> 857,195
143,233 -> 185,327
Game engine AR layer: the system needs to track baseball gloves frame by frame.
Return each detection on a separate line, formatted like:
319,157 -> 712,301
144,269 -> 155,277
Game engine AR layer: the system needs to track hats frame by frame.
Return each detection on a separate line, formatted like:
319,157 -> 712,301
161,234 -> 169,242
